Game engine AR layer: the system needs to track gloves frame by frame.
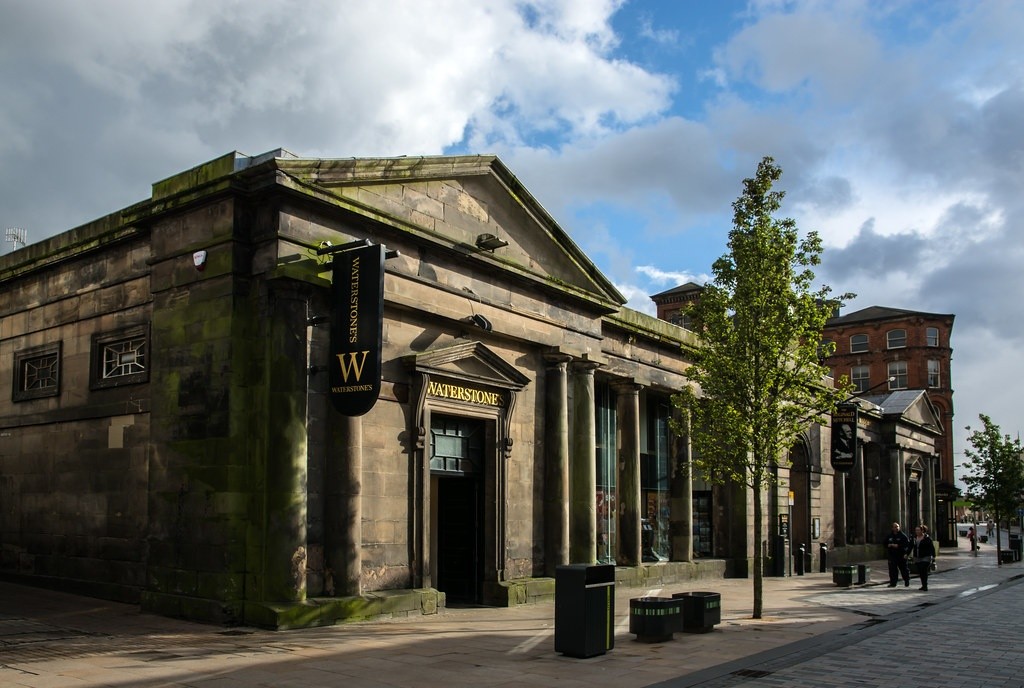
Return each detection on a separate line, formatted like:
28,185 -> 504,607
931,563 -> 938,572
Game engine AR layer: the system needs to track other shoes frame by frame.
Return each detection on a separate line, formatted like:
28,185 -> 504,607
905,580 -> 910,587
919,586 -> 925,590
924,587 -> 928,592
887,584 -> 897,588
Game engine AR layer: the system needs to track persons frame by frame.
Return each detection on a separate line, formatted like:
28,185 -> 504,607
967,527 -> 980,551
987,520 -> 995,537
832,424 -> 853,457
884,522 -> 910,587
904,525 -> 936,591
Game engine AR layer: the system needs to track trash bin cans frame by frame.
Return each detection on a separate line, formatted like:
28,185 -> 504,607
1009,533 -> 1023,561
555,564 -> 615,659
